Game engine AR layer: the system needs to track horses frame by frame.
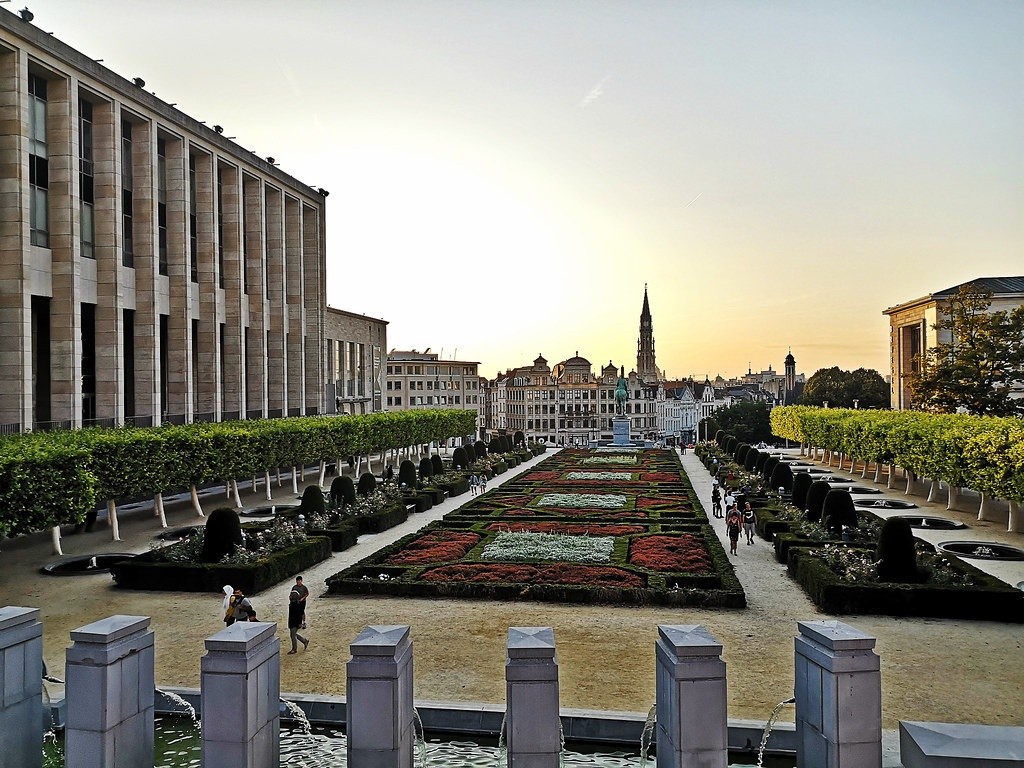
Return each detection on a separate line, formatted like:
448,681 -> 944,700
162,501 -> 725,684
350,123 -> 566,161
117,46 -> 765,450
615,389 -> 627,415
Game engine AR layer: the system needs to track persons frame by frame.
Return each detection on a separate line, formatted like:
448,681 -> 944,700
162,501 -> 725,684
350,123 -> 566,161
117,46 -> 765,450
726,512 -> 742,555
355,457 -> 363,474
248,610 -> 259,622
232,586 -> 252,621
291,576 -> 309,628
223,585 -> 235,626
743,502 -> 757,545
478,473 -> 487,494
382,466 -> 389,480
725,491 -> 736,523
469,473 -> 479,495
712,485 -> 718,515
680,442 -> 687,455
387,465 -> 394,480
286,593 -> 309,654
727,504 -> 741,548
715,493 -> 724,518
485,461 -> 498,476
348,455 -> 353,473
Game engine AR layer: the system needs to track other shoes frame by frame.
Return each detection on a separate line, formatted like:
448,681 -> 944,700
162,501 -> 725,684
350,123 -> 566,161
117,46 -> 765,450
730,550 -> 732,554
304,638 -> 309,649
734,553 -> 737,556
302,623 -> 307,629
747,541 -> 750,545
716,516 -> 724,519
750,538 -> 754,544
287,649 -> 297,654
